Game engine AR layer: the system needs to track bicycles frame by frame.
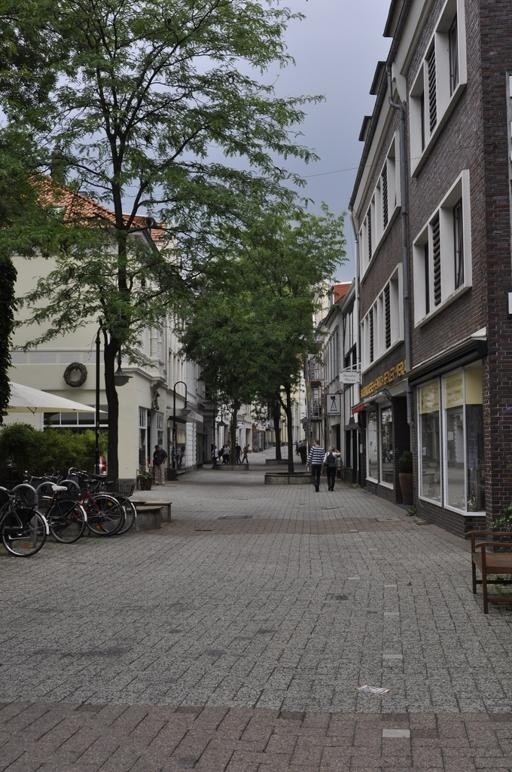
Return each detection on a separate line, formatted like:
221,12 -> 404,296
0,466 -> 138,557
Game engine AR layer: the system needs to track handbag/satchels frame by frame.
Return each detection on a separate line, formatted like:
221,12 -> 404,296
327,455 -> 335,462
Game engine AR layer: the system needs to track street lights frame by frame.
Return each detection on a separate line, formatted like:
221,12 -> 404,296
172,381 -> 190,469
95,322 -> 133,478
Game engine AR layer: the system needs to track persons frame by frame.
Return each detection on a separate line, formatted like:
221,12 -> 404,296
153,444 -> 168,487
295,439 -> 307,465
305,439 -> 326,494
323,446 -> 342,492
209,439 -> 250,471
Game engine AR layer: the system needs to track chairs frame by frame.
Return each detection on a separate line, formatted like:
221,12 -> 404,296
465,530 -> 512,614
